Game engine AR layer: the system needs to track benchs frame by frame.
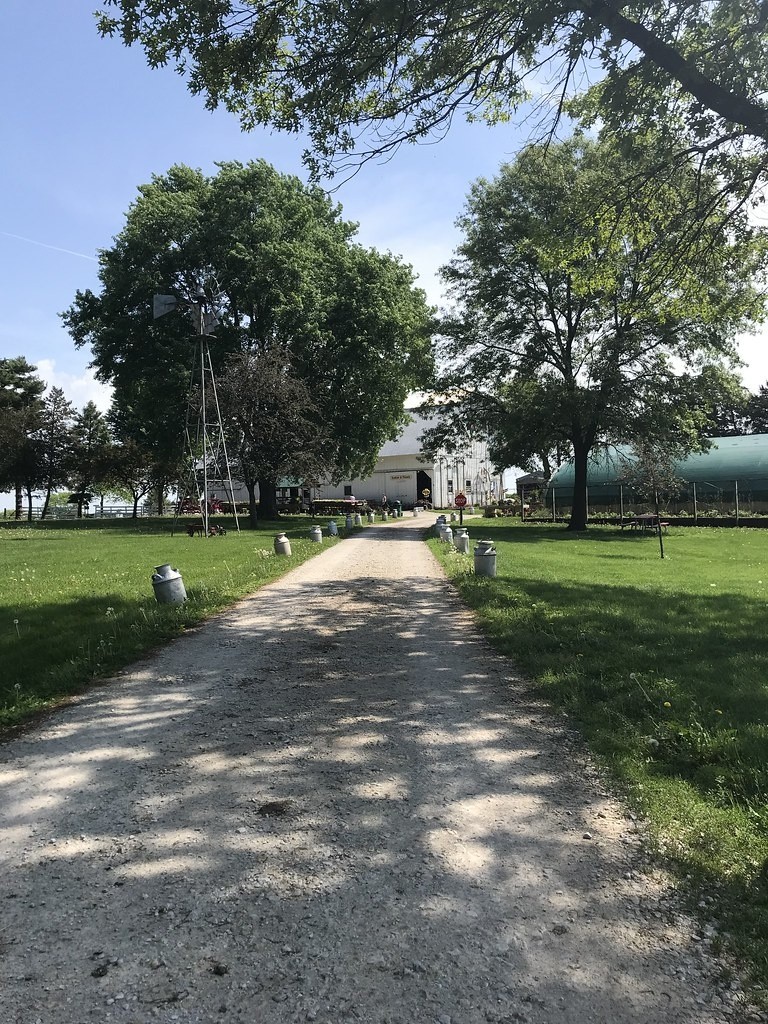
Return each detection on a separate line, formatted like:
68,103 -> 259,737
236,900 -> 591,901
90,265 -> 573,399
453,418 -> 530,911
620,522 -> 669,535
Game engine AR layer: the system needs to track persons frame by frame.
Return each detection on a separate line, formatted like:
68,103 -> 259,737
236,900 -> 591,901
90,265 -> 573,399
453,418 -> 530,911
381,494 -> 387,511
350,494 -> 355,499
421,488 -> 430,501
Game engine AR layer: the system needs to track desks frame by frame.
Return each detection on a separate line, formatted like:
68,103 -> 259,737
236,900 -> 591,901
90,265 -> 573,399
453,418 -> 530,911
630,515 -> 658,533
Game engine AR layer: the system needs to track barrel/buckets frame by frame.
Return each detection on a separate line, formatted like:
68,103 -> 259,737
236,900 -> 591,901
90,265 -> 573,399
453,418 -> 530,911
345,515 -> 352,529
437,514 -> 447,538
455,528 -> 470,557
310,525 -> 322,543
470,506 -> 474,514
151,561 -> 188,606
274,532 -> 291,556
440,524 -> 453,543
382,510 -> 388,520
413,508 -> 418,517
355,513 -> 361,526
368,512 -> 375,523
329,521 -> 339,535
393,508 -> 398,519
451,511 -> 457,522
473,541 -> 496,577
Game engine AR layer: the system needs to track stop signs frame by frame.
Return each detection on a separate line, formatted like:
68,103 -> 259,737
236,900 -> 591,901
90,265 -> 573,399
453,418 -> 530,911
455,494 -> 467,507
